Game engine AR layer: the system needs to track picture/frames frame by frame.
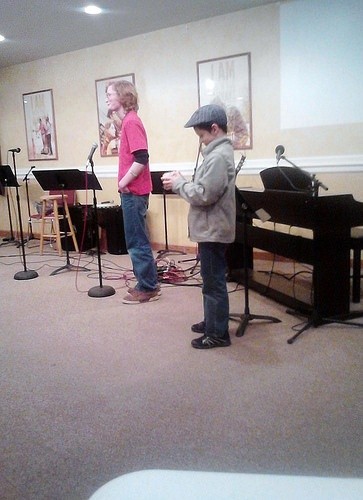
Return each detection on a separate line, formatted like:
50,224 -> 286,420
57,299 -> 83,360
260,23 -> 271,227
22,89 -> 58,161
95,73 -> 136,158
197,52 -> 254,150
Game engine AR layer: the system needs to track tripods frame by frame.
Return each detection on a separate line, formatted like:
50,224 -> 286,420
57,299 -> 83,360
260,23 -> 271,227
16,167 -> 56,248
0,165 -> 24,245
148,170 -> 186,260
279,155 -> 363,344
226,185 -> 283,337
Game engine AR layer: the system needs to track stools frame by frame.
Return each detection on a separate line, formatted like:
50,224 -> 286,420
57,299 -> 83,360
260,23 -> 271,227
351,228 -> 363,303
39,195 -> 80,257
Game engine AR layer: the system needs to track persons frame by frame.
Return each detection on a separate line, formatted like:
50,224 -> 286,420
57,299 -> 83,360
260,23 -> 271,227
32,118 -> 48,154
210,95 -> 248,145
100,108 -> 122,156
161,104 -> 235,349
43,117 -> 54,155
104,80 -> 161,303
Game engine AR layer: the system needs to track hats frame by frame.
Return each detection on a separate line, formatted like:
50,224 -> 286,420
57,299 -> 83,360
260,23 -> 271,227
184,104 -> 228,127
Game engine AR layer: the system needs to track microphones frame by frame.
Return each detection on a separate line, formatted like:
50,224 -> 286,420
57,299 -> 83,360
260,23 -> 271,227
274,144 -> 285,159
85,142 -> 98,168
8,147 -> 21,153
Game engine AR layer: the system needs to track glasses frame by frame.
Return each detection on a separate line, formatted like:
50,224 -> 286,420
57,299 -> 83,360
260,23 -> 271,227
104,92 -> 118,100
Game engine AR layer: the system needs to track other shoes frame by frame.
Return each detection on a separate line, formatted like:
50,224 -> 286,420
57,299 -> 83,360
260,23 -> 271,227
126,283 -> 161,296
122,290 -> 159,304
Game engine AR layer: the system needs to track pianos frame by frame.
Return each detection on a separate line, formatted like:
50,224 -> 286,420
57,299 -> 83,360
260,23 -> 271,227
229,186 -> 363,328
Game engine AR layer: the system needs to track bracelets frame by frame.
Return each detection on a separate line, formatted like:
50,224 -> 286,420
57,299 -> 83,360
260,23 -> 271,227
128,169 -> 138,178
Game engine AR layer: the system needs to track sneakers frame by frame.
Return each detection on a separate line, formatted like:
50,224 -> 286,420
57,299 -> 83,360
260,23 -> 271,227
191,320 -> 206,334
191,332 -> 231,350
